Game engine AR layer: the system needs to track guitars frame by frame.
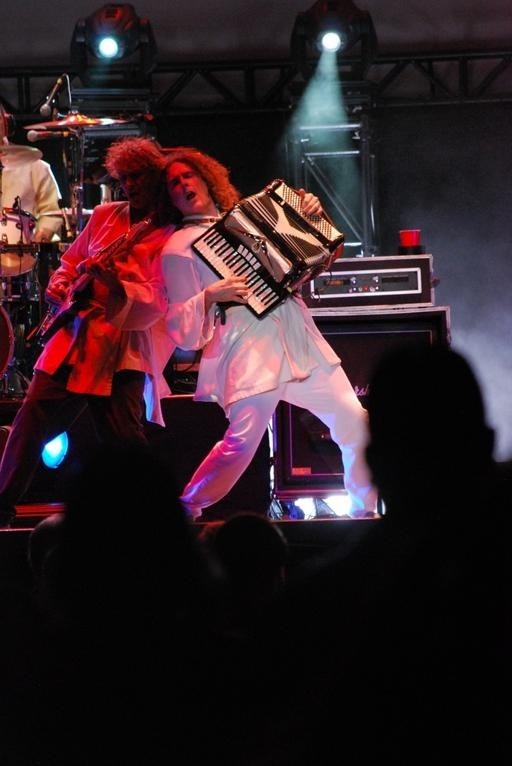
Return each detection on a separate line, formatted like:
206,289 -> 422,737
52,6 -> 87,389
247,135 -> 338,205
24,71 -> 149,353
36,212 -> 155,349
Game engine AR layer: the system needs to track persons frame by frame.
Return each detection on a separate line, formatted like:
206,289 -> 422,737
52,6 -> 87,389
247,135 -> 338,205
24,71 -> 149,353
1,338 -> 510,766
161,147 -> 376,522
1,138 -> 176,525
1,104 -> 63,244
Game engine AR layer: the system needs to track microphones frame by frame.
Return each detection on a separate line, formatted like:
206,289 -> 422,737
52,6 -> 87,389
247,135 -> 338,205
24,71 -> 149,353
40,74 -> 65,118
12,196 -> 20,209
27,130 -> 73,142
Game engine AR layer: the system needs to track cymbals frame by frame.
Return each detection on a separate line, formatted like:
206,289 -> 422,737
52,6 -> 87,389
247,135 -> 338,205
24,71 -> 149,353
42,207 -> 93,218
0,208 -> 37,224
0,252 -> 36,278
23,115 -> 129,131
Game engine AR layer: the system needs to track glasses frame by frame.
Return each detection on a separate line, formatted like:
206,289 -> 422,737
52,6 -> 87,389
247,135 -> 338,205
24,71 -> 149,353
120,172 -> 139,184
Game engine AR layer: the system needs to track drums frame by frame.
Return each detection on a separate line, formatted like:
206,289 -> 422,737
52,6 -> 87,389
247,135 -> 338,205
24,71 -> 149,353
0,307 -> 15,380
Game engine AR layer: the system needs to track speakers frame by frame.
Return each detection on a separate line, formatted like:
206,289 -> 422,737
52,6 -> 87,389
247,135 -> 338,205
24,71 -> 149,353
274,304 -> 451,495
160,395 -> 271,515
